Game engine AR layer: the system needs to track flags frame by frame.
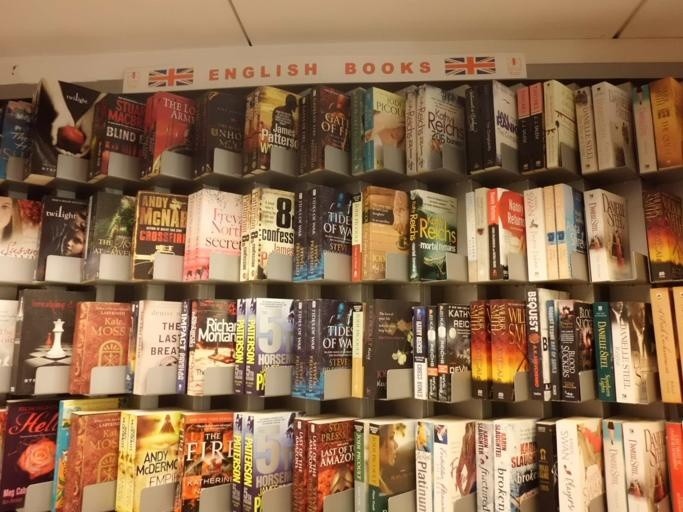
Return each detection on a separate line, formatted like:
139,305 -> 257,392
444,57 -> 495,73
149,69 -> 192,86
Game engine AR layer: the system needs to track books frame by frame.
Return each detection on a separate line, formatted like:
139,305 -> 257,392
34,196 -> 90,286
523,182 -> 589,282
68,301 -> 130,395
592,299 -> 658,403
406,189 -> 458,287
171,414 -> 232,511
0,195 -> 46,286
644,187 -> 681,282
0,398 -> 59,512
648,287 -> 682,404
467,300 -> 531,402
230,413 -> 295,510
523,287 -> 596,404
49,400 -> 119,511
410,303 -> 474,402
239,188 -> 295,280
175,299 -> 237,395
233,298 -> 295,398
290,300 -> 352,400
650,77 -> 681,171
191,92 -> 243,181
0,101 -> 34,182
631,84 -> 658,175
534,413 -> 606,511
351,300 -> 415,400
123,300 -> 181,396
11,287 -> 92,395
466,186 -> 527,282
182,188 -> 240,282
347,89 -> 406,178
141,94 -> 197,192
404,84 -> 467,179
129,190 -> 189,282
347,186 -> 410,283
572,81 -> 637,174
21,78 -> 102,187
81,192 -> 134,283
291,417 -> 356,511
88,90 -> 146,189
115,409 -> 177,511
297,87 -> 352,178
471,418 -> 540,512
600,418 -> 682,510
515,81 -> 578,172
0,298 -> 16,393
461,81 -> 521,176
413,414 -> 477,512
352,418 -> 416,511
582,188 -> 634,283
240,87 -> 301,181
292,188 -> 351,283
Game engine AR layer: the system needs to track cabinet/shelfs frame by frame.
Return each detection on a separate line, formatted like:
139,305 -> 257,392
0,62 -> 677,511
